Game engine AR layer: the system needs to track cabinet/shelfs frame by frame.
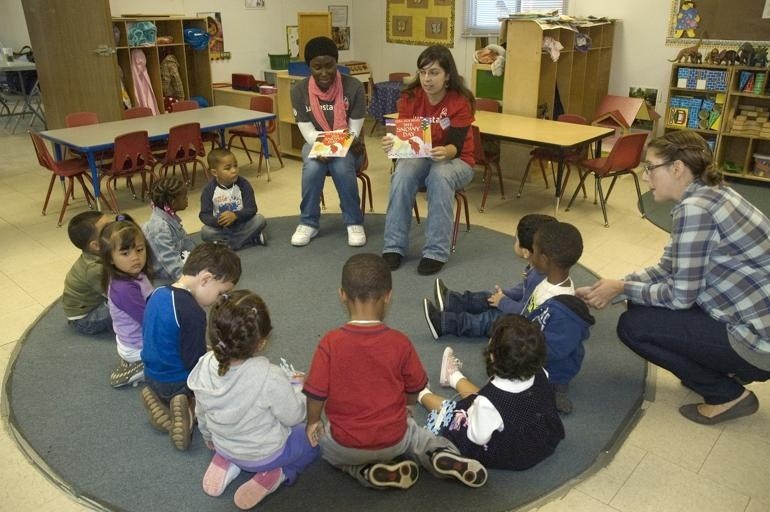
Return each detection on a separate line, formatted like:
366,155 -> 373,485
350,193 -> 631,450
470,63 -> 504,113
112,16 -> 214,115
661,63 -> 770,185
499,19 -> 616,183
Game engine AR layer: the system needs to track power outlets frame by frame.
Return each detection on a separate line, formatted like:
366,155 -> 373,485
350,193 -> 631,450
3,48 -> 14,63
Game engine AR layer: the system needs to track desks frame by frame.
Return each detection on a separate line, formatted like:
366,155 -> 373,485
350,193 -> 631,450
0,55 -> 47,134
382,110 -> 614,217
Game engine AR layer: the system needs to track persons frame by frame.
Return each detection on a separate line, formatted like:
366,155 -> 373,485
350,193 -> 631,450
183,287 -> 321,510
416,310 -> 566,471
57,210 -> 113,341
380,44 -> 476,276
516,221 -> 596,414
421,213 -> 561,339
98,211 -> 155,389
140,241 -> 240,450
207,15 -> 224,53
198,148 -> 268,253
574,129 -> 770,428
300,251 -> 489,490
289,36 -> 371,247
139,177 -> 201,286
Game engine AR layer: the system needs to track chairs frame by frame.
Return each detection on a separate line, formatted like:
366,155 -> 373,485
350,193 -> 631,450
27,95 -> 283,226
369,82 -> 404,137
564,134 -> 646,229
23,86 -> 47,131
0,83 -> 11,116
516,115 -> 587,204
387,159 -> 470,254
368,71 -> 376,104
389,73 -> 410,82
471,98 -> 505,211
320,128 -> 373,218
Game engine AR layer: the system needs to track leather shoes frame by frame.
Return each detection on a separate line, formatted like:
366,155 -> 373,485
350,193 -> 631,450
676,368 -> 757,423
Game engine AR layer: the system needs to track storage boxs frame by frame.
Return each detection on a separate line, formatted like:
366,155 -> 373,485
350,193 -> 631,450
752,153 -> 770,179
264,70 -> 288,85
288,62 -> 312,76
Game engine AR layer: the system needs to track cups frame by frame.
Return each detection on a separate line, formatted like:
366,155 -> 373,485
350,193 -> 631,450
2,47 -> 14,66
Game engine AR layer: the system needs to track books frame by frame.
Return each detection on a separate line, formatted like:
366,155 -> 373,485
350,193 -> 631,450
308,132 -> 356,157
385,118 -> 433,159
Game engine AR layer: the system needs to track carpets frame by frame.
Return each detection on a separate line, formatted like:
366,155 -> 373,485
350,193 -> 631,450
639,178 -> 770,236
2,213 -> 647,512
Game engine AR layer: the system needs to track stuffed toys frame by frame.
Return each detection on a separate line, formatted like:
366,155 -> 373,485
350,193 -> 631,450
673,0 -> 701,38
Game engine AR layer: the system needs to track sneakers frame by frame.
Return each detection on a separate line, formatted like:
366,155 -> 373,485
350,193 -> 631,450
108,352 -> 147,388
427,448 -> 488,489
439,346 -> 461,388
417,256 -> 443,275
252,232 -> 266,244
167,394 -> 195,450
344,223 -> 367,247
382,251 -> 401,269
291,223 -> 320,245
201,448 -> 240,498
142,385 -> 170,433
357,459 -> 420,489
422,297 -> 444,340
233,465 -> 285,510
434,277 -> 449,313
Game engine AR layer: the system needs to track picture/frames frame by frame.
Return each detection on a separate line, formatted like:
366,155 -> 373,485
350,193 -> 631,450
286,25 -> 300,59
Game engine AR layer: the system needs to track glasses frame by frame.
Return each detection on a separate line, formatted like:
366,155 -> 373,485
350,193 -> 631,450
643,160 -> 670,172
415,68 -> 442,77
